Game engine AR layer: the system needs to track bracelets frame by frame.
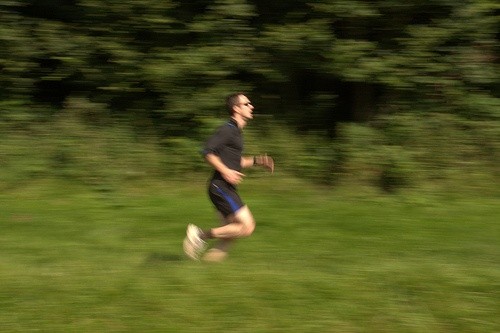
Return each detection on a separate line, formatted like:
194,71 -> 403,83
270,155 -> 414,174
252,154 -> 257,167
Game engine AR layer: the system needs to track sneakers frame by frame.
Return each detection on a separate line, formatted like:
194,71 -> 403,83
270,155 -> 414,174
181,222 -> 204,262
201,247 -> 228,262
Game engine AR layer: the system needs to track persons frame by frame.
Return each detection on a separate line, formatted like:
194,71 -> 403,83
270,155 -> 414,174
181,89 -> 279,263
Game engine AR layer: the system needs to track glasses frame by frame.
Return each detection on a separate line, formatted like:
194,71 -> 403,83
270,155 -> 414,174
237,102 -> 252,107
